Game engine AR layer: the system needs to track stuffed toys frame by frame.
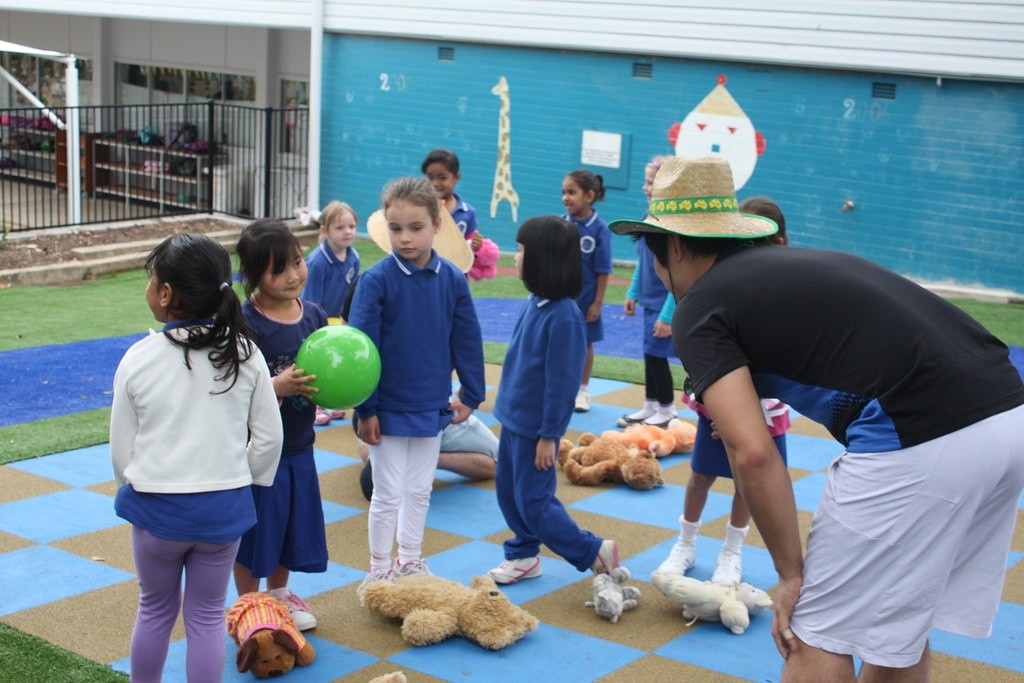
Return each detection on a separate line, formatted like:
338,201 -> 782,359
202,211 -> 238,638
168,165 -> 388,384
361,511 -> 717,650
650,569 -> 773,635
464,233 -> 497,280
584,566 -> 641,624
558,433 -> 665,490
365,575 -> 538,650
604,417 -> 697,457
226,591 -> 316,677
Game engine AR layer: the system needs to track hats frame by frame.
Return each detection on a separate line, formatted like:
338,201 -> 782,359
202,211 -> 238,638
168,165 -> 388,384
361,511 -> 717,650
608,153 -> 779,239
367,198 -> 474,276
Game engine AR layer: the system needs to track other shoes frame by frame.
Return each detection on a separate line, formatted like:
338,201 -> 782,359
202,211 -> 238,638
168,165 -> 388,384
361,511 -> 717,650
311,402 -> 345,425
360,458 -> 374,502
639,411 -> 677,428
618,410 -> 656,428
576,392 -> 590,412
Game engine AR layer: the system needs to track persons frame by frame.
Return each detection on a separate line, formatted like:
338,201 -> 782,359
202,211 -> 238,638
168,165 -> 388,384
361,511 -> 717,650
108,231 -> 285,682
352,384 -> 501,500
485,216 -> 617,584
617,155 -> 681,431
421,151 -> 483,283
608,156 -> 1024,683
228,222 -> 328,631
656,195 -> 789,585
297,201 -> 362,427
557,171 -> 613,411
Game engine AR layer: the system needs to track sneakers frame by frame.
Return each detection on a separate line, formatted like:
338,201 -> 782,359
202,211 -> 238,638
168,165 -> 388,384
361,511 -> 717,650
589,540 -> 616,574
392,558 -> 434,579
654,547 -> 695,581
489,553 -> 541,583
267,588 -> 319,633
357,565 -> 392,603
713,551 -> 742,586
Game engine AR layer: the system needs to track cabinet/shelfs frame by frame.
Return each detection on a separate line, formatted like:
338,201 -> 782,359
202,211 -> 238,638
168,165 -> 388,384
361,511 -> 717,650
56,132 -> 109,189
0,127 -> 55,182
90,139 -> 226,209
